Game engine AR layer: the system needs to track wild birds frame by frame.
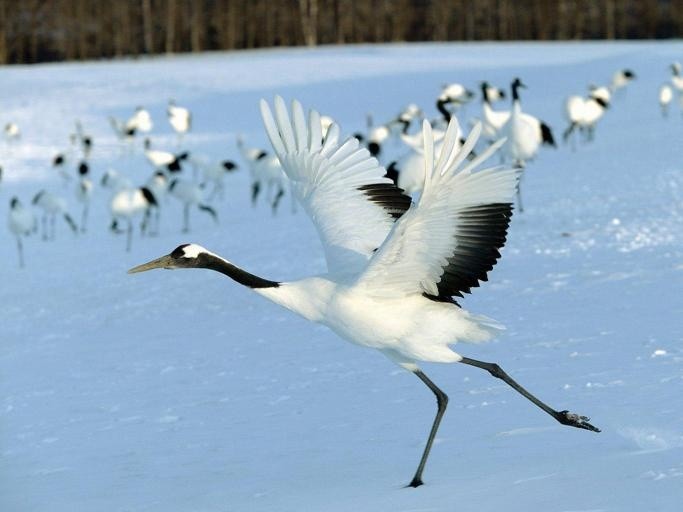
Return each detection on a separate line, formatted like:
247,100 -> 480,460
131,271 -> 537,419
660,85 -> 672,108
610,70 -> 636,91
670,63 -> 681,76
126,96 -> 599,489
354,79 -> 558,212
0,100 -> 296,268
562,85 -> 613,140
672,77 -> 683,89
